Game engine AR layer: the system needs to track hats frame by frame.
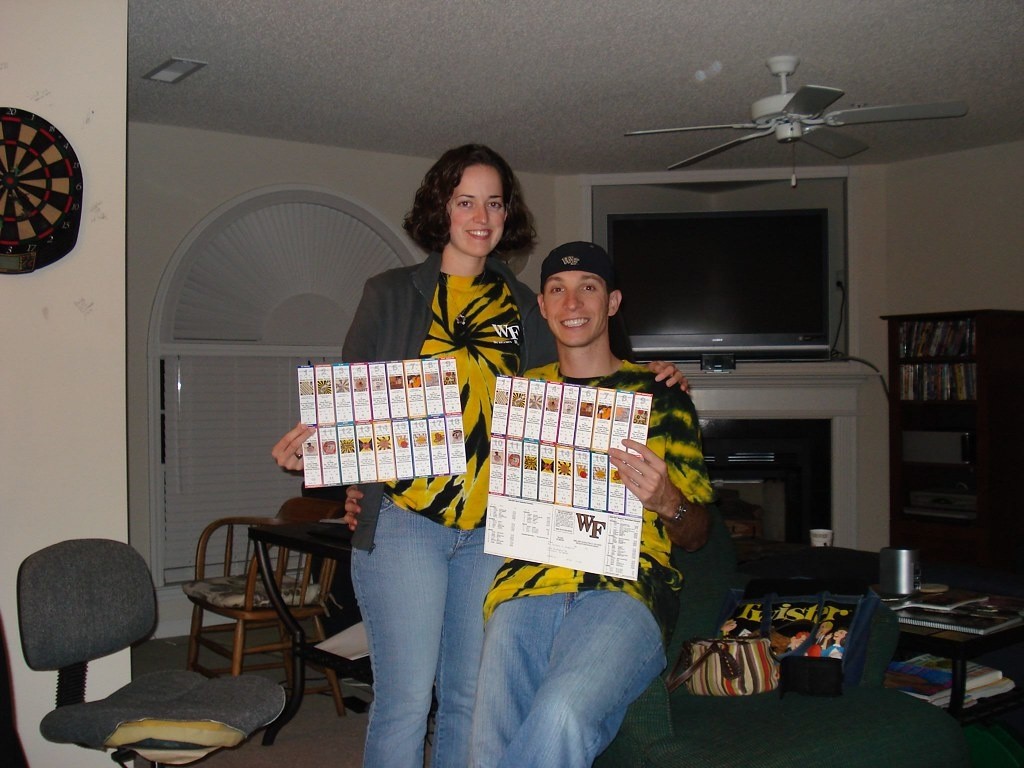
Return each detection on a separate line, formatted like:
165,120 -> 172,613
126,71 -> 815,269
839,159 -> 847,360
541,241 -> 615,287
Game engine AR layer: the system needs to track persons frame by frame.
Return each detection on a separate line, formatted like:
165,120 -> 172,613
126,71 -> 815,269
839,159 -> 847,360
273,138 -> 692,767
345,243 -> 714,767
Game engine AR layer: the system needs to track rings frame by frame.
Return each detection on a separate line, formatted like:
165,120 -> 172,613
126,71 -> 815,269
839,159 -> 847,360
294,451 -> 303,459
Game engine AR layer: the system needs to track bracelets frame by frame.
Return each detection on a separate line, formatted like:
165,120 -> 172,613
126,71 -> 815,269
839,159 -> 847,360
663,489 -> 688,523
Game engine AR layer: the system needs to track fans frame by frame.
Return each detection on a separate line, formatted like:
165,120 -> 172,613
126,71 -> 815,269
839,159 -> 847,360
622,54 -> 969,190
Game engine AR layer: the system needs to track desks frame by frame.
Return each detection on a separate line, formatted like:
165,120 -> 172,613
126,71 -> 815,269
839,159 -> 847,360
885,587 -> 1024,724
243,516 -> 378,746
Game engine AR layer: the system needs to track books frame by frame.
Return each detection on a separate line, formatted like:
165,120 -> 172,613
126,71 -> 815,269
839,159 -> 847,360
884,588 -> 1022,716
891,321 -> 981,407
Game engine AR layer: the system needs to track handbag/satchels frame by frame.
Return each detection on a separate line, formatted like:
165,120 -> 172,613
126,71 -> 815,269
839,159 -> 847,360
666,634 -> 779,697
717,588 -> 899,697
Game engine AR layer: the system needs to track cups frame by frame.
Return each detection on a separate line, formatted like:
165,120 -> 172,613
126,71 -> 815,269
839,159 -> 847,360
810,529 -> 832,547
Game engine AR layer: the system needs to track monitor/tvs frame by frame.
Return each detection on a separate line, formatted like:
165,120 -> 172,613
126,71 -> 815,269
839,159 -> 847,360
607,208 -> 830,372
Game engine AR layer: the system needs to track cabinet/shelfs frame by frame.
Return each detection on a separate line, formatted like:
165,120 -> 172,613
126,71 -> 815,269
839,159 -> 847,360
881,308 -> 1024,571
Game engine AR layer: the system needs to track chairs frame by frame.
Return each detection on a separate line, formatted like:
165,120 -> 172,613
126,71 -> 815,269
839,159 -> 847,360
186,495 -> 351,719
17,538 -> 287,768
